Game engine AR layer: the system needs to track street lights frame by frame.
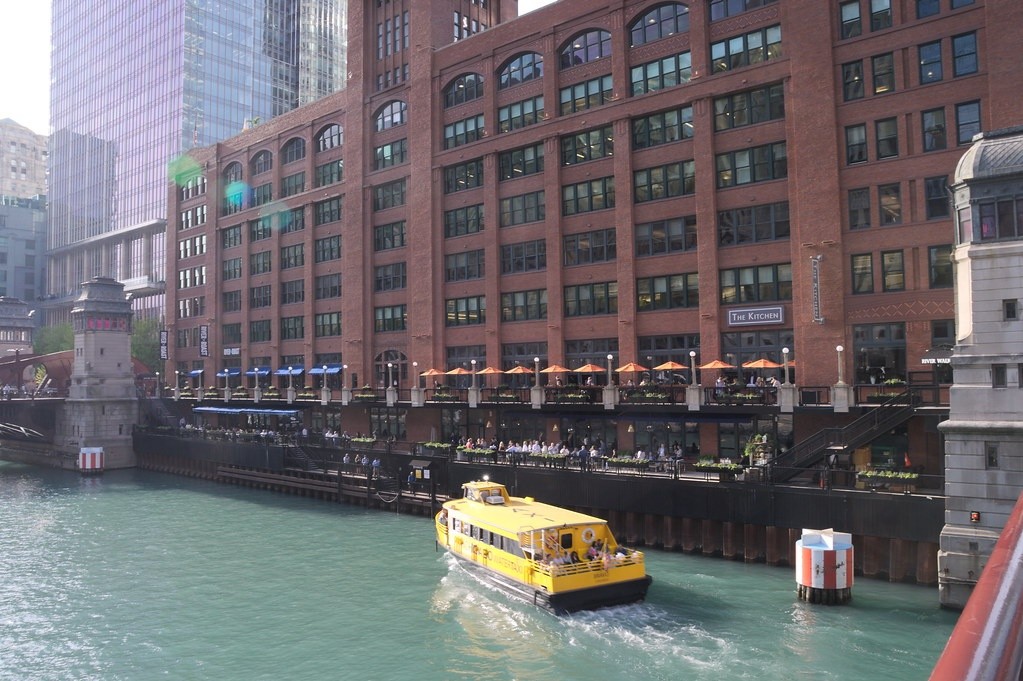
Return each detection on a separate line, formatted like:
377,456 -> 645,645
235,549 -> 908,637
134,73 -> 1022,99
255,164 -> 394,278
388,363 -> 393,387
288,366 -> 292,388
322,365 -> 327,388
254,368 -> 259,388
413,362 -> 418,387
156,371 -> 160,389
197,370 -> 202,387
836,345 -> 844,382
224,368 -> 229,387
606,354 -> 614,385
533,357 -> 541,386
782,346 -> 790,383
343,365 -> 348,388
689,351 -> 697,385
174,371 -> 179,387
471,360 -> 476,388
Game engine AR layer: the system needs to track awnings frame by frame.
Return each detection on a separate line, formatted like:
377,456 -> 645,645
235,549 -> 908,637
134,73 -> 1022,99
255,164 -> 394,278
185,370 -> 204,378
216,363 -> 343,378
192,405 -> 305,416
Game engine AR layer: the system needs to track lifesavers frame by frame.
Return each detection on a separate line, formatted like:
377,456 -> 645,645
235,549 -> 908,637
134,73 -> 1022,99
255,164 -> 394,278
581,528 -> 595,543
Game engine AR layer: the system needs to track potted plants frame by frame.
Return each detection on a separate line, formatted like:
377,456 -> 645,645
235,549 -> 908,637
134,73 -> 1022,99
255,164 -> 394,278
204,392 -> 220,398
431,395 -> 459,401
693,460 -> 743,474
629,392 -> 670,402
355,394 -> 379,401
868,393 -> 919,403
463,448 -> 495,458
263,392 -> 282,398
857,471 -> 921,485
716,394 -> 764,402
232,392 -> 248,398
607,458 -> 649,468
556,394 -> 588,401
489,394 -> 519,402
424,442 -> 450,451
298,393 -> 319,400
529,452 -> 567,463
352,437 -> 375,446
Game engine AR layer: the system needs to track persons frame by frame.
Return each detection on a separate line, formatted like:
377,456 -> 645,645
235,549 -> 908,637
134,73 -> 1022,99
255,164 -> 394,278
542,551 -> 580,576
637,443 -> 682,472
756,376 -> 780,393
715,375 -> 725,394
355,455 -> 361,476
556,376 -> 646,386
0,382 -> 10,396
287,426 -> 377,446
491,436 -> 606,470
343,453 -> 351,475
450,432 -> 487,450
362,455 -> 369,472
373,458 -> 380,476
435,380 -> 441,394
180,418 -> 274,437
588,539 -> 626,561
630,549 -> 639,564
408,472 -> 415,493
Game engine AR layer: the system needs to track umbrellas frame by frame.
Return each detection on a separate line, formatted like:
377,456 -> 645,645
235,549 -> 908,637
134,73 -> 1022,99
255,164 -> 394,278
419,358 -> 781,376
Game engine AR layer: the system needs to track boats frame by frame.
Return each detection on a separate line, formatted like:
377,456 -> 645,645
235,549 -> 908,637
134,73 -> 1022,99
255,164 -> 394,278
434,480 -> 654,615
